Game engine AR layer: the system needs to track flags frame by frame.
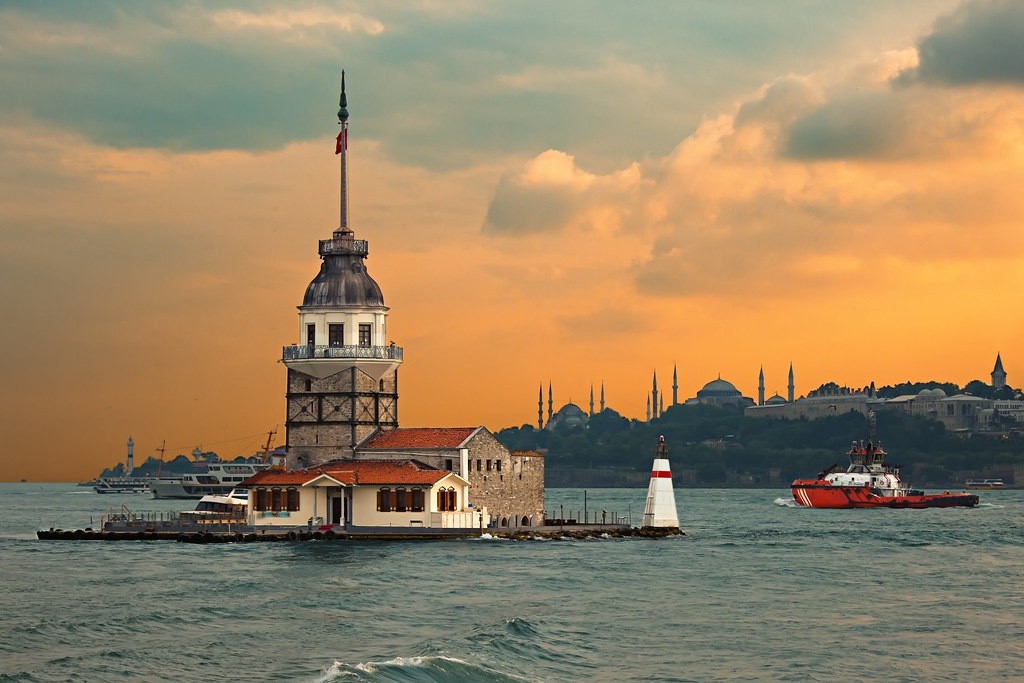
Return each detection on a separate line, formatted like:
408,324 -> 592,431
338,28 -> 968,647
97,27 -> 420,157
335,129 -> 347,155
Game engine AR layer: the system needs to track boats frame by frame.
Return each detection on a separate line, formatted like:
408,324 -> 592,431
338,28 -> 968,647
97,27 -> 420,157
790,408 -> 979,509
179,461 -> 272,499
144,471 -> 204,501
95,474 -> 159,495
